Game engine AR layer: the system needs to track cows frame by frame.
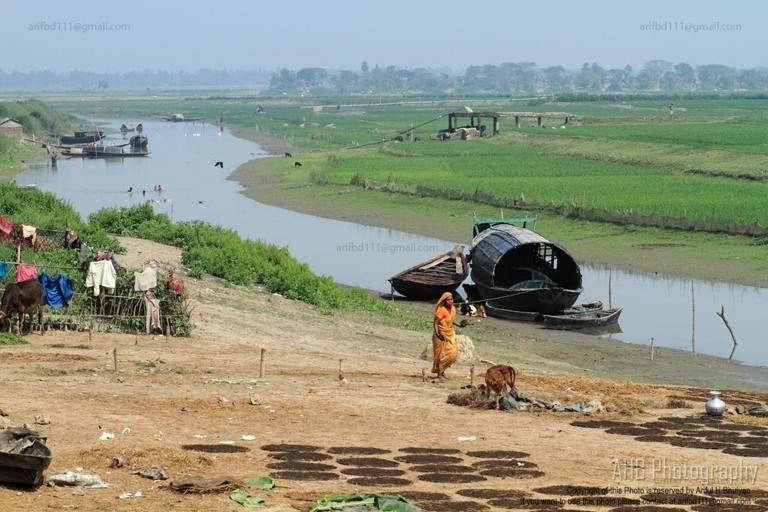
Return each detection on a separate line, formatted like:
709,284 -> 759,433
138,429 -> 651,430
285,153 -> 292,158
0,279 -> 45,335
295,162 -> 302,168
485,364 -> 520,410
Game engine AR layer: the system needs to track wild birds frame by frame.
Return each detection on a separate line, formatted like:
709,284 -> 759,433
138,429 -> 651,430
215,162 -> 223,168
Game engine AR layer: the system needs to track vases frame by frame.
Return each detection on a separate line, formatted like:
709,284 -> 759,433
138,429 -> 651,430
706,390 -> 726,416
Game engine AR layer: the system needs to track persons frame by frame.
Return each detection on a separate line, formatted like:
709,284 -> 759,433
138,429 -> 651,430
431,291 -> 466,381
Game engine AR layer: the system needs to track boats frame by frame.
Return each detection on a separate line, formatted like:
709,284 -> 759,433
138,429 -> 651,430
60,123 -> 151,156
0,424 -> 53,486
387,244 -> 470,302
161,114 -> 201,121
466,208 -> 624,329
